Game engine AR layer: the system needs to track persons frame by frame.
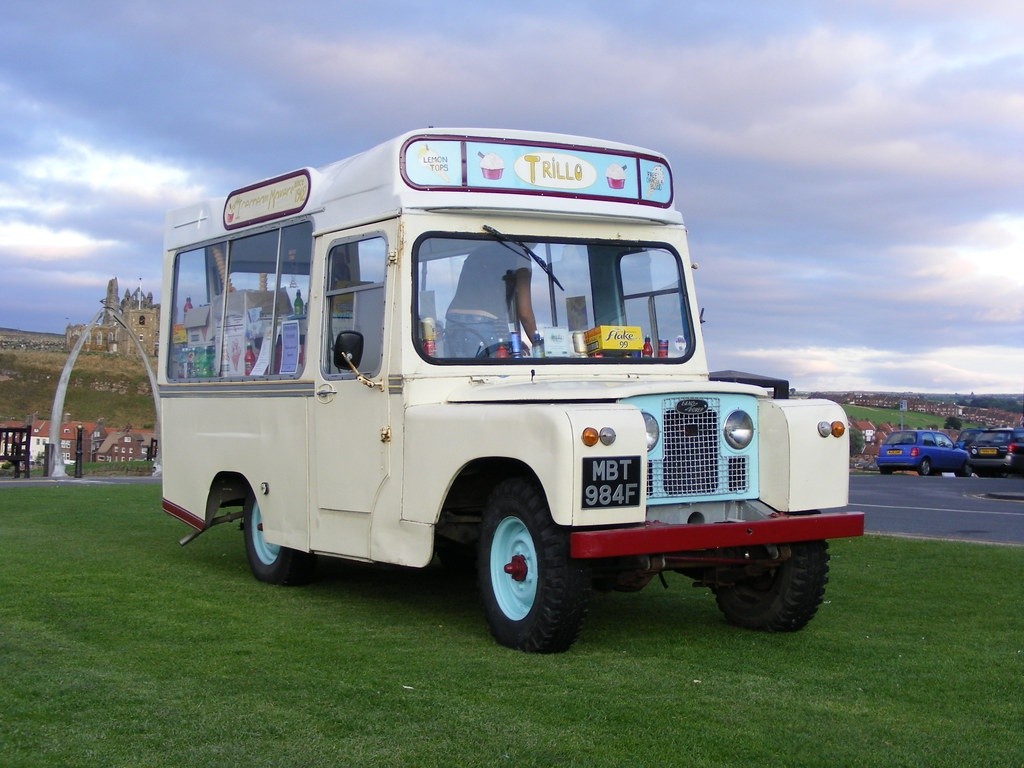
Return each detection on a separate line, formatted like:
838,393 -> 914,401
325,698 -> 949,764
443,240 -> 540,359
1021,412 -> 1024,427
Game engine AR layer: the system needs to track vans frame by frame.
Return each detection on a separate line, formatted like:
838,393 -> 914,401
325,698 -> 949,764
153,125 -> 866,654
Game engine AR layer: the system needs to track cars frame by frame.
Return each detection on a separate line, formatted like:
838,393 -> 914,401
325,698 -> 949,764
955,427 -> 1024,478
877,430 -> 972,477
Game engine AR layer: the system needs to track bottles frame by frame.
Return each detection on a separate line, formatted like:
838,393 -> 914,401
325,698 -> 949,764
294,289 -> 303,314
245,343 -> 255,376
184,296 -> 193,322
532,330 -> 545,358
496,339 -> 508,358
642,336 -> 652,358
173,345 -> 214,379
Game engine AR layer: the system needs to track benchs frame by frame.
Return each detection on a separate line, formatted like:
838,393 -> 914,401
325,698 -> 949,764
0,425 -> 32,480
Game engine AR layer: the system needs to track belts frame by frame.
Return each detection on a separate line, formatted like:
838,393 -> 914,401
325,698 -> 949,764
446,308 -> 499,325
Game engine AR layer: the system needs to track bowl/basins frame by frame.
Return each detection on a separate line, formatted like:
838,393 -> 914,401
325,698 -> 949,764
482,166 -> 504,180
607,176 -> 625,189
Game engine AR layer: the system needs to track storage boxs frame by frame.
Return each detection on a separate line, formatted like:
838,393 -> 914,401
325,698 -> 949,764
172,290 -> 295,376
582,325 -> 645,354
330,278 -> 375,313
